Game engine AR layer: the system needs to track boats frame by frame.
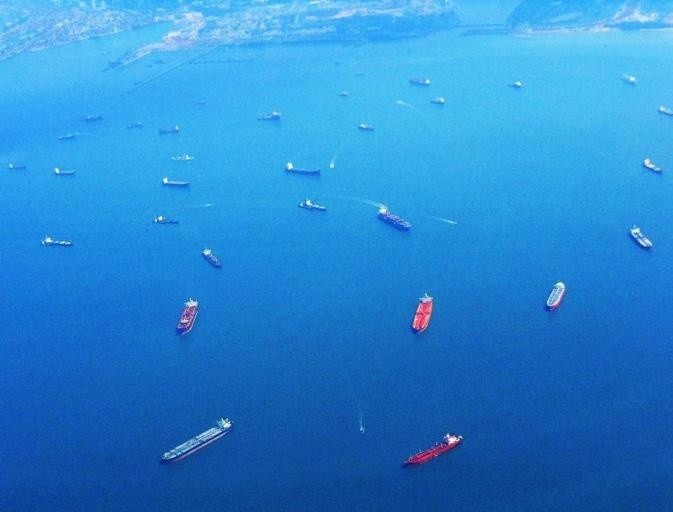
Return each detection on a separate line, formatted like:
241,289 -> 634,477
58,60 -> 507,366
409,77 -> 446,106
643,158 -> 663,175
162,178 -> 190,186
40,238 -> 72,247
201,248 -> 221,267
152,215 -> 179,225
170,154 -> 195,162
658,106 -> 673,116
58,133 -> 74,141
8,163 -> 27,170
629,224 -> 653,248
620,73 -> 636,84
54,168 -> 76,175
376,208 -> 412,231
84,115 -> 103,122
286,163 -> 322,176
297,199 -> 326,211
509,81 -> 523,89
358,123 -> 376,132
158,126 -> 179,134
126,122 -> 144,130
256,111 -> 281,121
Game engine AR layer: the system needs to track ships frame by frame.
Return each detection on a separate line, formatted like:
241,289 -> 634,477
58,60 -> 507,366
412,292 -> 434,334
173,297 -> 200,338
545,282 -> 565,310
399,432 -> 462,468
156,418 -> 234,466
